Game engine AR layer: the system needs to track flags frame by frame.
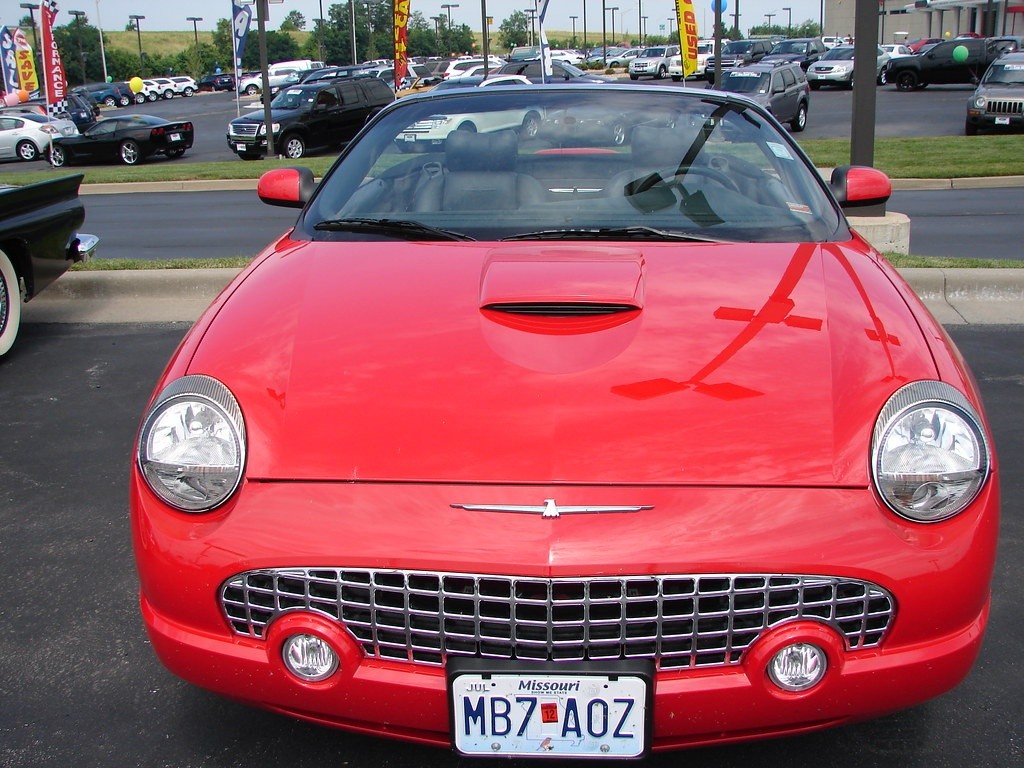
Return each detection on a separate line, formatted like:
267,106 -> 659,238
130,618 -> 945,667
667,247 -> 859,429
232,0 -> 252,99
0,26 -> 19,95
41,0 -> 66,105
675,0 -> 698,79
393,0 -> 410,90
536,0 -> 553,84
12,27 -> 39,95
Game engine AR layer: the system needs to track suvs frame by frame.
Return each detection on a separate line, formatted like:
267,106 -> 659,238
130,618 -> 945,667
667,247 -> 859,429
224,76 -> 398,162
964,50 -> 1024,135
703,60 -> 811,139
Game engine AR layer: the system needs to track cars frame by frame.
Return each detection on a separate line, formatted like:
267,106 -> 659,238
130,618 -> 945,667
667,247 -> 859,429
124,80 -> 1005,764
392,72 -> 547,154
0,27 -> 1024,169
42,113 -> 195,168
0,112 -> 81,163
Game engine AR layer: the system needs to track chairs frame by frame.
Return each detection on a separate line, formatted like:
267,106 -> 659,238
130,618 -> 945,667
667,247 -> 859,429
606,122 -> 733,202
415,129 -> 555,212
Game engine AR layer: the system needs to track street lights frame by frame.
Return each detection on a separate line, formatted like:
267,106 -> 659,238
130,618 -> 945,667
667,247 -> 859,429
441,4 -> 460,57
568,15 -> 578,49
641,16 -> 649,46
20,3 -> 45,97
68,10 -> 88,85
782,7 -> 792,39
485,16 -> 493,55
765,14 -> 776,35
667,18 -> 675,43
728,13 -> 742,41
605,7 -> 619,46
430,16 -> 443,57
128,15 -> 146,80
524,9 -> 538,47
186,17 -> 203,45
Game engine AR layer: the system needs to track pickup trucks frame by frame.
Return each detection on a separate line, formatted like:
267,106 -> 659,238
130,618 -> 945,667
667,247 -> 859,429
883,36 -> 1024,94
0,173 -> 101,363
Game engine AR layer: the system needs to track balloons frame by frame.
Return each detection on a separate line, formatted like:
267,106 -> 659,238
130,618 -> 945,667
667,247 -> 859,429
6,93 -> 18,107
711,0 -> 727,14
945,32 -> 951,38
106,76 -> 112,83
216,68 -> 221,74
130,77 -> 143,94
953,46 -> 969,64
18,90 -> 29,103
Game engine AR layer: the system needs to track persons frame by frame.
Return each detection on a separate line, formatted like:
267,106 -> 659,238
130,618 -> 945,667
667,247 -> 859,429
847,34 -> 854,44
905,37 -> 910,46
